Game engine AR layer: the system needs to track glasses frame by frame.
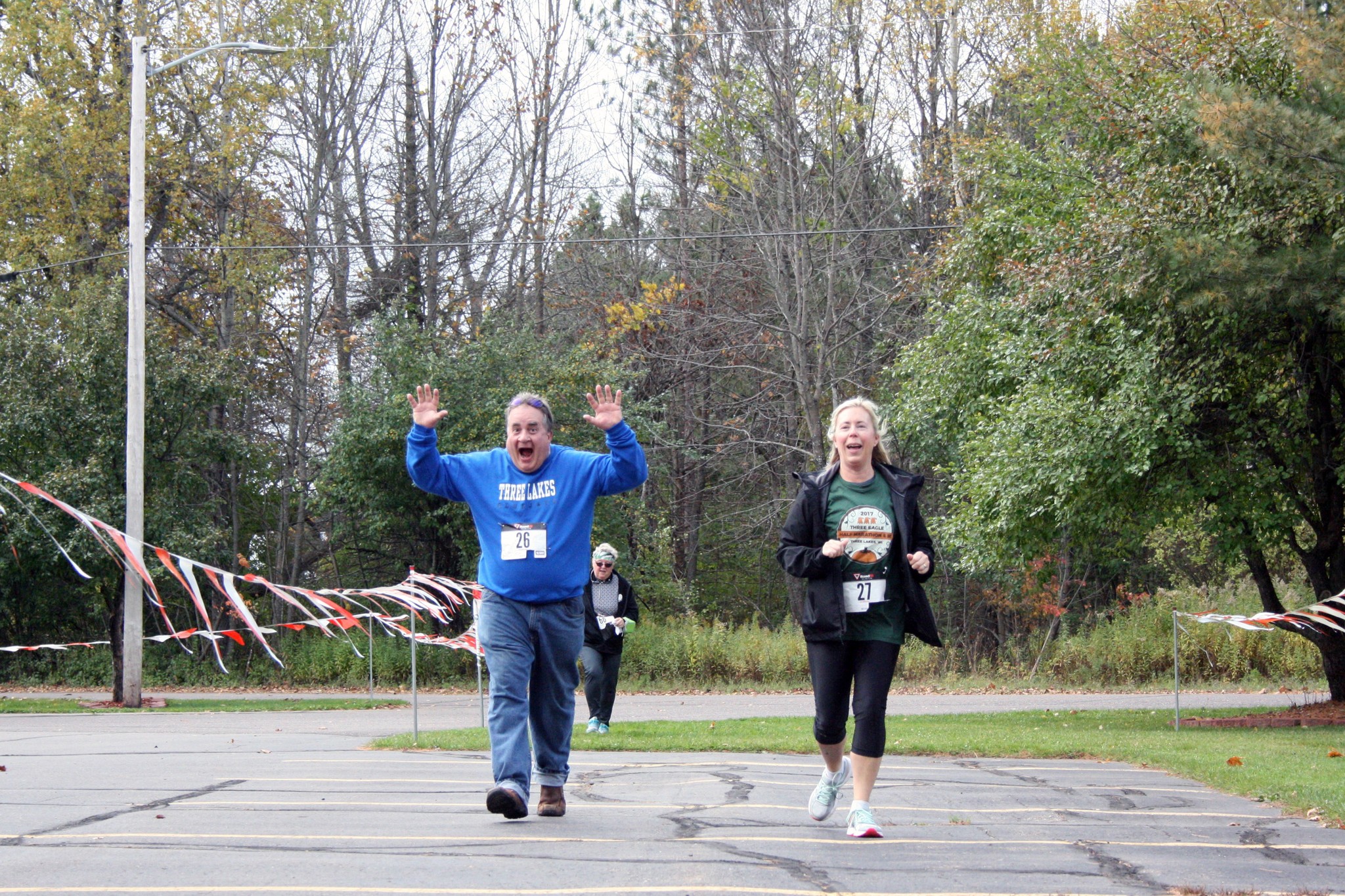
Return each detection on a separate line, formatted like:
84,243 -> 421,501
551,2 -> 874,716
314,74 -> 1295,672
594,559 -> 613,567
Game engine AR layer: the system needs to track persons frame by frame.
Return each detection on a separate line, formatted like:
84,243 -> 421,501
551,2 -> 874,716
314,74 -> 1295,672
579,543 -> 639,733
776,396 -> 943,839
404,384 -> 647,820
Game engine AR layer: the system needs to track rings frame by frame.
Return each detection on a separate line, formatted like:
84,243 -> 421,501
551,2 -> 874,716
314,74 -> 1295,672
923,564 -> 926,568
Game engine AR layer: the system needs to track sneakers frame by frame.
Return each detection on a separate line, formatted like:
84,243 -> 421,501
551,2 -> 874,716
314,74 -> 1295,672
586,716 -> 600,733
599,723 -> 609,734
846,808 -> 884,838
536,785 -> 566,816
486,786 -> 528,819
808,756 -> 852,821
